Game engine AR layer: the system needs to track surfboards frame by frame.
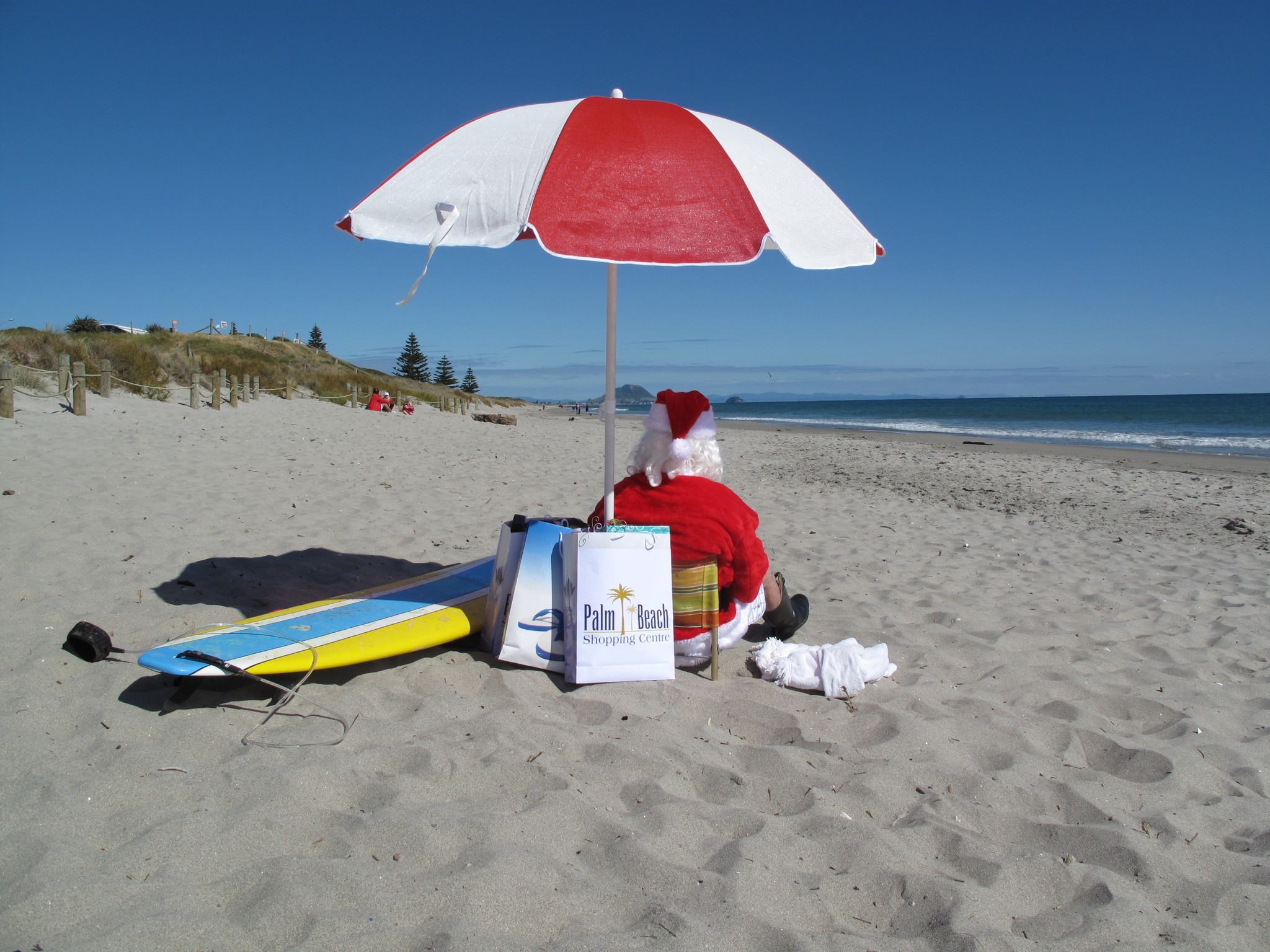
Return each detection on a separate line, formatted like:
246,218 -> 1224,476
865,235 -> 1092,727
138,554 -> 499,677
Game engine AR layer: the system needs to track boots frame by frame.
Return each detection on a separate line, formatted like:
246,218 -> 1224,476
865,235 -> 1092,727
760,570 -> 811,642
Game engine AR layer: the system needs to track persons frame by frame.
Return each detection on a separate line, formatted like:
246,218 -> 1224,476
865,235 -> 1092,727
543,405 -> 545,410
560,403 -> 588,416
587,388 -> 809,668
367,388 -> 397,413
382,392 -> 394,413
402,399 -> 414,415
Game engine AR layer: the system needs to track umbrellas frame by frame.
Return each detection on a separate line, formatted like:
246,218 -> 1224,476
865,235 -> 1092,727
334,89 -> 883,522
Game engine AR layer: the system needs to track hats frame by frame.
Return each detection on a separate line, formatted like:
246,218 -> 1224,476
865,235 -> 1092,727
640,389 -> 717,459
383,392 -> 389,396
405,398 -> 412,401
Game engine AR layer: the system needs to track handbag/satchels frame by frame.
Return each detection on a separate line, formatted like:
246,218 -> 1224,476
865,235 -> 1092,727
558,523 -> 676,685
478,512 -> 595,676
